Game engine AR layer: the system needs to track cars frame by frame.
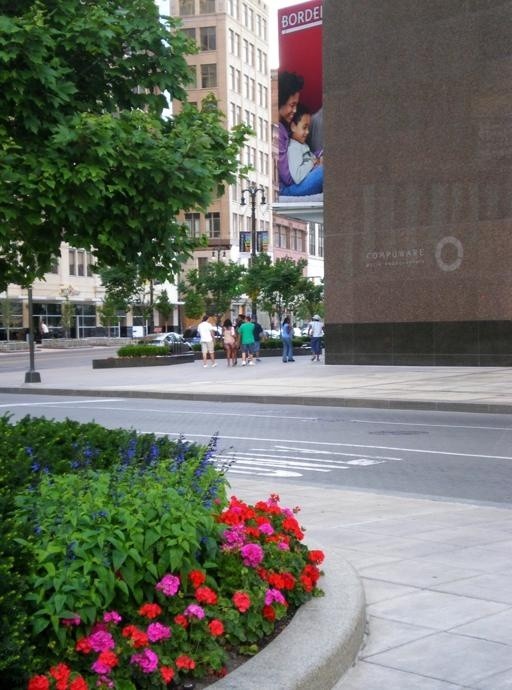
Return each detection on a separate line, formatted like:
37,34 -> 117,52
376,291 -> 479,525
138,327 -> 224,352
299,323 -> 311,337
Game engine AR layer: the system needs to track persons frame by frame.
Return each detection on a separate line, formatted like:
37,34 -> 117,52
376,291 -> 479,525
196,315 -> 263,369
307,315 -> 324,361
281,316 -> 298,363
277,70 -> 323,198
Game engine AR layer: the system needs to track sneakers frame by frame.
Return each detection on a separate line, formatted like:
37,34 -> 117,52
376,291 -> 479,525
203,359 -> 261,368
311,356 -> 320,362
283,360 -> 295,363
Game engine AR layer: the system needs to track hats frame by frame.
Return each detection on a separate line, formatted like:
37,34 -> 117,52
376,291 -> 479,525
312,314 -> 321,320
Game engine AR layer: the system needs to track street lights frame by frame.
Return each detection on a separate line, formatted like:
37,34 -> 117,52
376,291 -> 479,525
240,181 -> 267,315
